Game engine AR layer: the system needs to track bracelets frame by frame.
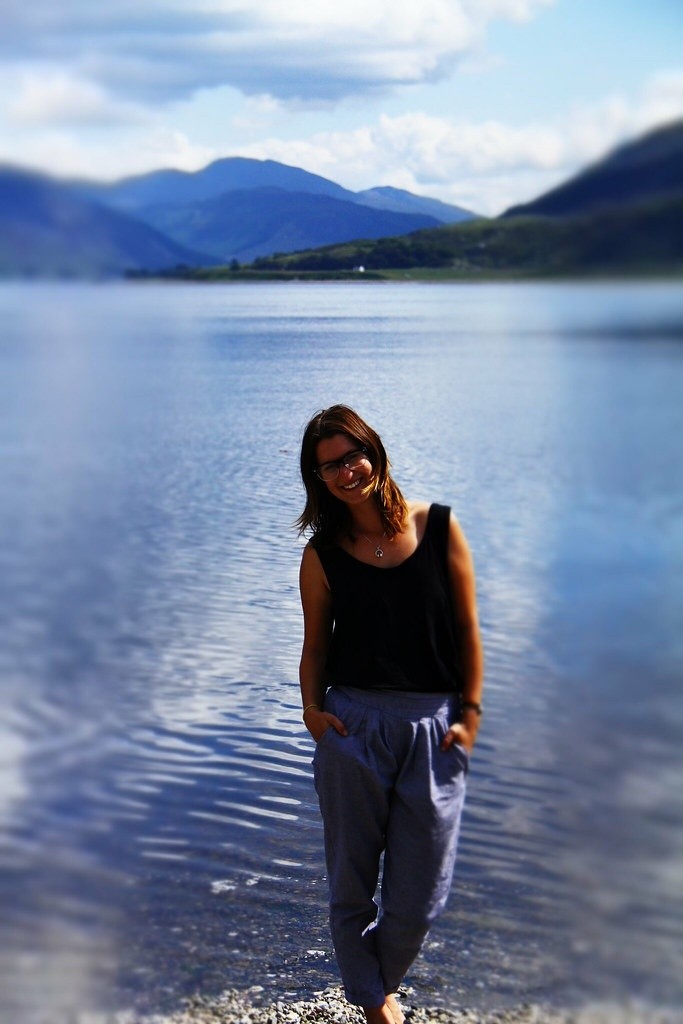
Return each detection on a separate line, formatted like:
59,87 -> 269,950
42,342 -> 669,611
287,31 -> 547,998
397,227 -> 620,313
462,701 -> 483,716
303,704 -> 317,713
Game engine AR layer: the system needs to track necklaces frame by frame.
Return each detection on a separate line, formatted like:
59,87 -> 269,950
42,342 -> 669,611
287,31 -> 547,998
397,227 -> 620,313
361,530 -> 386,558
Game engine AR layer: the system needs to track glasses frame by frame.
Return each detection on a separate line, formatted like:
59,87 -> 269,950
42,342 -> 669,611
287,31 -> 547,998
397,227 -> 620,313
314,445 -> 366,482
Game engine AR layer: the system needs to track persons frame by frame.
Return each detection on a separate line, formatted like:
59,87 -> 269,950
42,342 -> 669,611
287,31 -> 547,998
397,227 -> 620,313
298,404 -> 483,1024
